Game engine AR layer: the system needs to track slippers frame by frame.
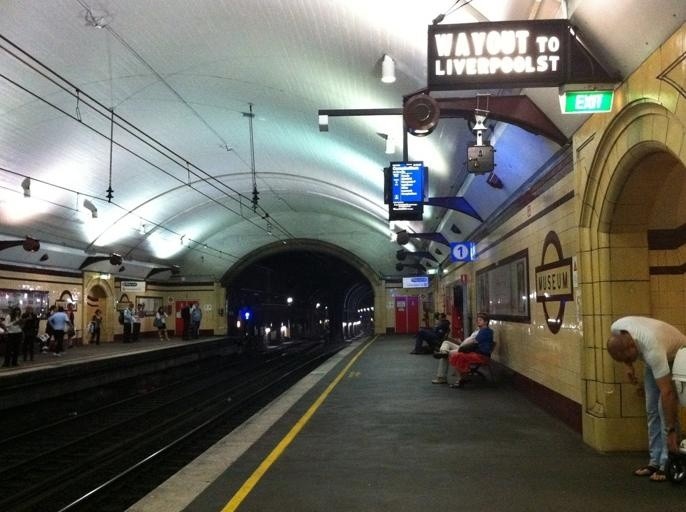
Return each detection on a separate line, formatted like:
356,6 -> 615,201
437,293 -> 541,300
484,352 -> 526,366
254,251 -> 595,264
633,465 -> 657,476
649,470 -> 667,481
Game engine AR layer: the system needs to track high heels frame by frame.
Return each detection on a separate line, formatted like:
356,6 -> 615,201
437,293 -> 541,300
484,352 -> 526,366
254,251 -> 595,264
449,379 -> 465,388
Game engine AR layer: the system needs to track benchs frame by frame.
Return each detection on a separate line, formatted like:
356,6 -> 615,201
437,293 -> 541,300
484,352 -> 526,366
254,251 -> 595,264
452,341 -> 497,385
420,327 -> 450,352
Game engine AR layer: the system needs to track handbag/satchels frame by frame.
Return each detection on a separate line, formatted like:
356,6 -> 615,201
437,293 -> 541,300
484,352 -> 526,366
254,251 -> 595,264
153,318 -> 162,327
457,342 -> 477,353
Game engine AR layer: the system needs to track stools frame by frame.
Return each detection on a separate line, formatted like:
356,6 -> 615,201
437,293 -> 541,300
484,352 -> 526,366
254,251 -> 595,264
199,329 -> 213,336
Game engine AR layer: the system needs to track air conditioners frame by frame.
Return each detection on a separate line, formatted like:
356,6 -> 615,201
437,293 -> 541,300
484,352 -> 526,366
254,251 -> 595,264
167,296 -> 176,303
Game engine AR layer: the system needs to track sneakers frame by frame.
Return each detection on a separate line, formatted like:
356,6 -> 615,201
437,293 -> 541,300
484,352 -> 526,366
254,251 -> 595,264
433,350 -> 448,359
432,377 -> 448,384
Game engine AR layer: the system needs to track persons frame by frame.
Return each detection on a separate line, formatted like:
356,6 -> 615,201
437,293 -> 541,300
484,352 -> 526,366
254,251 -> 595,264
448,314 -> 494,388
607,316 -> 686,481
432,312 -> 496,384
0,301 -> 202,370
411,313 -> 451,354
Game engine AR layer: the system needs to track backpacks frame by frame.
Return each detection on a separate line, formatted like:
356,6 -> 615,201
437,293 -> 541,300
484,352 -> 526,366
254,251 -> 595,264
118,310 -> 124,324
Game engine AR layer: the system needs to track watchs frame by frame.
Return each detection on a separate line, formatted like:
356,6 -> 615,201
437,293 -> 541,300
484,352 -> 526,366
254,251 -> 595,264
664,427 -> 675,436
